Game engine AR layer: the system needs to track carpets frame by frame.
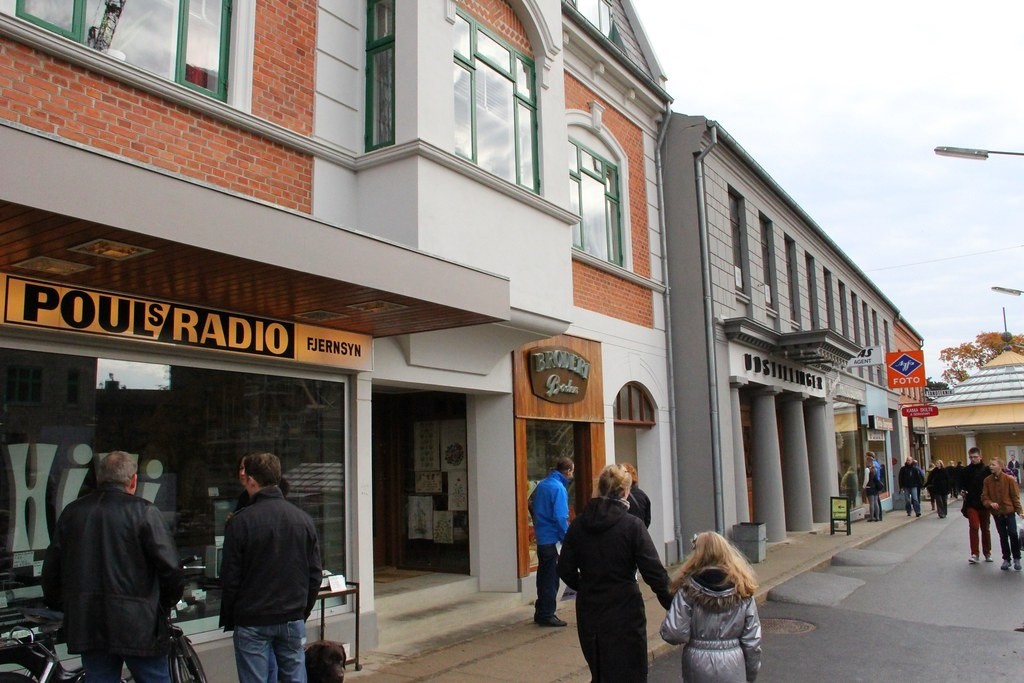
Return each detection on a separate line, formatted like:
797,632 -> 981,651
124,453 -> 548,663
374,566 -> 423,584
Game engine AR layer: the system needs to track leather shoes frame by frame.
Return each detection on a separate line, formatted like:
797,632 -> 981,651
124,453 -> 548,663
540,615 -> 568,628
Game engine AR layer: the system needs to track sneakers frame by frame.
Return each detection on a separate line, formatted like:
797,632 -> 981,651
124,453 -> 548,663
968,554 -> 979,563
1014,559 -> 1022,570
986,557 -> 994,562
1001,559 -> 1012,570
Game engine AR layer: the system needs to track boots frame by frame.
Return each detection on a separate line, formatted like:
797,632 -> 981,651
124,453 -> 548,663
932,502 -> 936,510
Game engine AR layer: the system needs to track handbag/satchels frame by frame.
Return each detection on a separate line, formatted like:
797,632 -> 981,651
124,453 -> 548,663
1017,517 -> 1024,551
566,559 -> 599,596
877,480 -> 884,490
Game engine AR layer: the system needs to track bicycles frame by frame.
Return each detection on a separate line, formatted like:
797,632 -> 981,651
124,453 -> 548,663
0,553 -> 211,683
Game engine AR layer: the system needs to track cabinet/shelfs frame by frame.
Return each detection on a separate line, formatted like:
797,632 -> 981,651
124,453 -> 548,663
316,580 -> 361,672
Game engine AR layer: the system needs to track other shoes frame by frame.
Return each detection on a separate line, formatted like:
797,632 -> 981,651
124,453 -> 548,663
867,519 -> 880,522
938,513 -> 946,518
916,512 -> 921,517
907,512 -> 911,516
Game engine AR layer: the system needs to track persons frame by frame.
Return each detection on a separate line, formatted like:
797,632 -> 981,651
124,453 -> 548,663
865,452 -> 884,521
528,457 -> 574,626
40,450 -> 185,683
621,462 -> 651,579
913,460 -> 925,505
556,465 -> 674,683
981,456 -> 1024,570
1008,455 -> 1020,485
958,447 -> 993,563
841,466 -> 857,508
927,460 -> 964,518
659,531 -> 762,683
863,456 -> 879,522
880,464 -> 886,492
221,454 -> 323,683
899,457 -> 921,518
226,455 -> 255,512
